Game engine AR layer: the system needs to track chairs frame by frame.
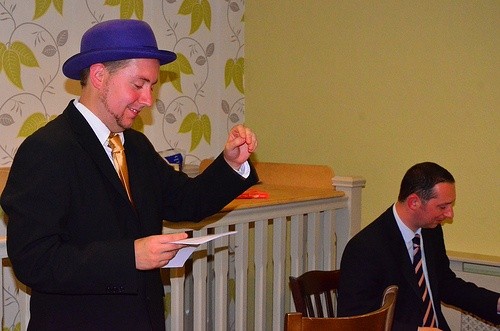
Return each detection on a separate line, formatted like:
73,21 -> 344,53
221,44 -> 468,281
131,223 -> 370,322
283,284 -> 399,331
288,268 -> 340,319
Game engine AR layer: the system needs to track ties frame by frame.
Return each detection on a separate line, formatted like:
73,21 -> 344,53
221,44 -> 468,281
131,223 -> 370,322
412,233 -> 437,327
107,132 -> 133,205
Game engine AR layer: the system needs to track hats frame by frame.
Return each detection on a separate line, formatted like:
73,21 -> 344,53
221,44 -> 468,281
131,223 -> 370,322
62,19 -> 177,80
